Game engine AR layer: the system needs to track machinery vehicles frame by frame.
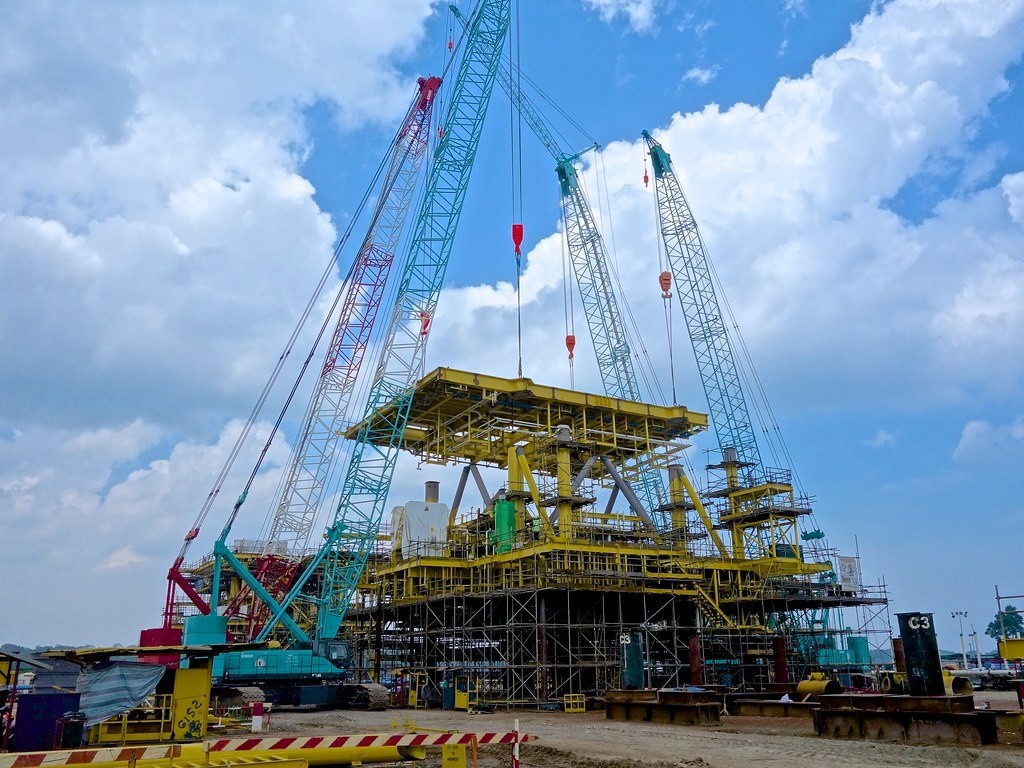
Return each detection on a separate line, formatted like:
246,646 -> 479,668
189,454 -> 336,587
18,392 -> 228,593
133,0 -> 874,716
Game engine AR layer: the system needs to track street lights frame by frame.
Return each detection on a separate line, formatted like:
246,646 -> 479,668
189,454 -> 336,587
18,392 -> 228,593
951,611 -> 969,671
